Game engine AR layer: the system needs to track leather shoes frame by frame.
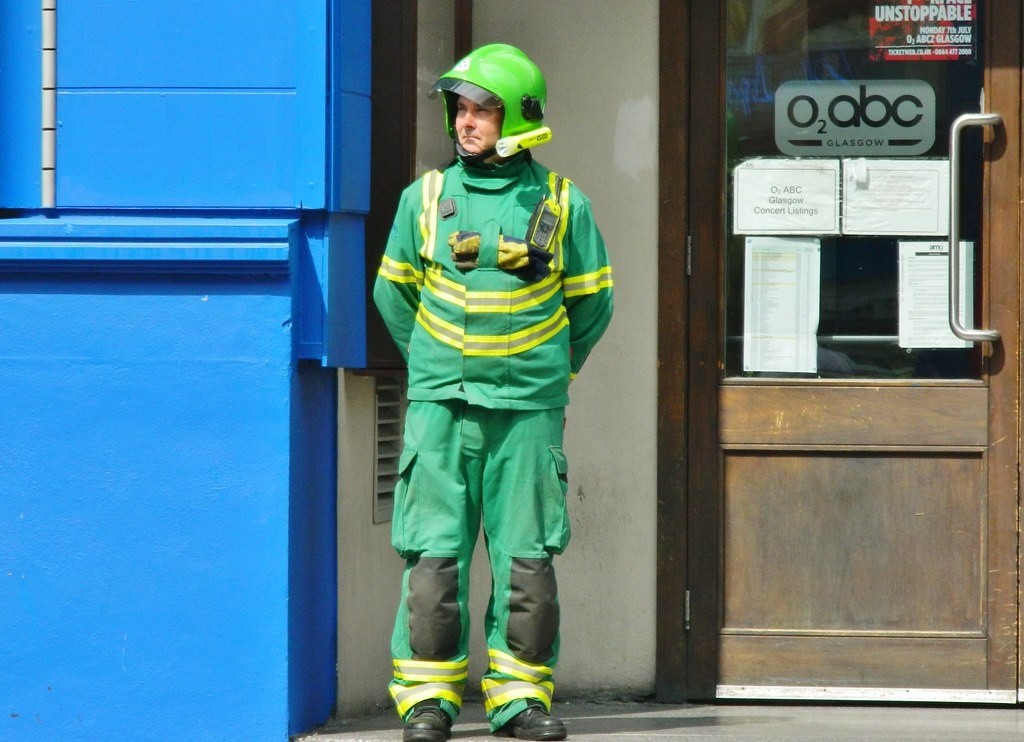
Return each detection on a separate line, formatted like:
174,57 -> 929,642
505,708 -> 567,739
401,700 -> 451,740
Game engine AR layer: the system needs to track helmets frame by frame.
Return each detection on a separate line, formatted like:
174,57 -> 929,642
440,42 -> 553,158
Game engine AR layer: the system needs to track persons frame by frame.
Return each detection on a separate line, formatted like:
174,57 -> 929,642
373,43 -> 614,742
745,297 -> 857,379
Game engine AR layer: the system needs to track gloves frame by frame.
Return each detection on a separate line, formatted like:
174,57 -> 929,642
449,229 -> 554,284
